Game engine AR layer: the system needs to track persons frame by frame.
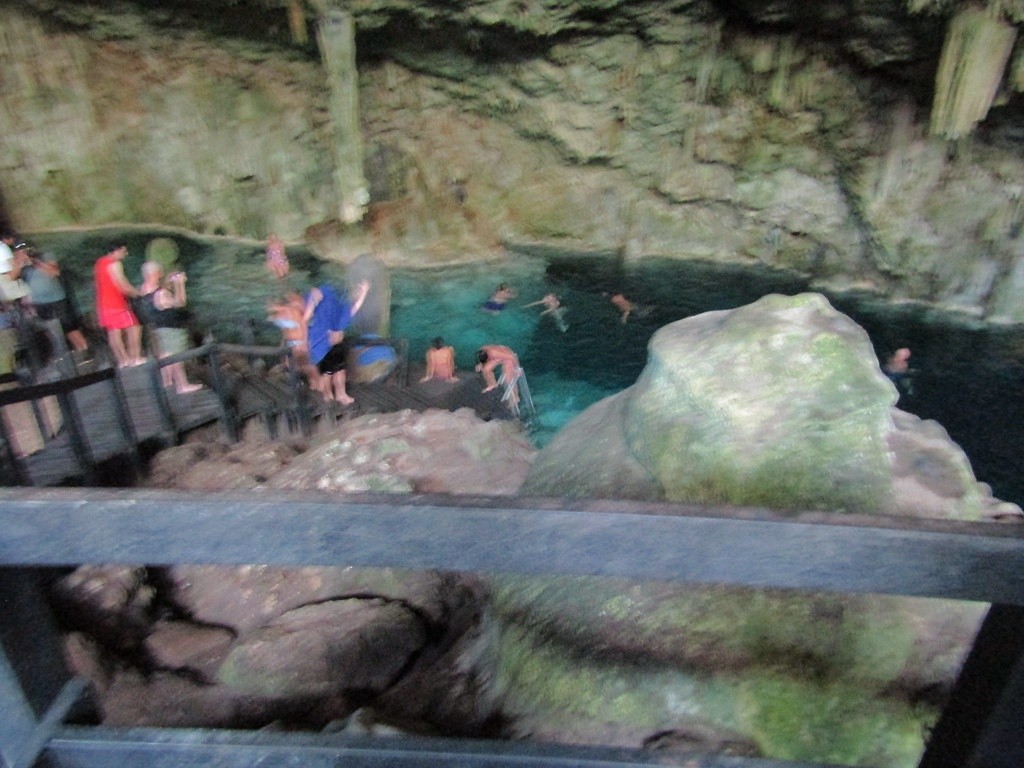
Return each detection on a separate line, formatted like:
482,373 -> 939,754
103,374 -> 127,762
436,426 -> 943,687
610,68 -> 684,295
480,282 -> 517,316
602,291 -> 631,324
519,291 -> 560,316
0,226 -> 371,409
475,344 -> 520,409
418,336 -> 459,385
885,347 -> 911,374
